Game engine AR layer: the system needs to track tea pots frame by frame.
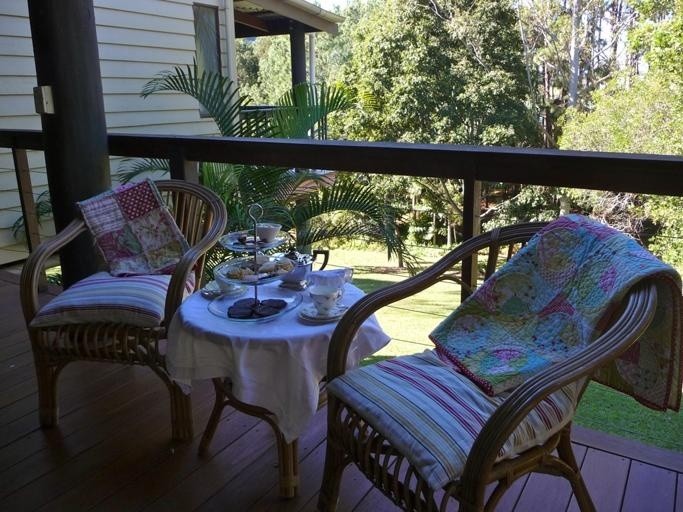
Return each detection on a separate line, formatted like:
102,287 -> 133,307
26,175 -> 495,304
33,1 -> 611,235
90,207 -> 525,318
276,247 -> 329,283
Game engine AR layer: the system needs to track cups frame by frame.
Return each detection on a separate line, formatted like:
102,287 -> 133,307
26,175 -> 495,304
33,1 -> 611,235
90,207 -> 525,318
308,286 -> 343,315
253,223 -> 282,242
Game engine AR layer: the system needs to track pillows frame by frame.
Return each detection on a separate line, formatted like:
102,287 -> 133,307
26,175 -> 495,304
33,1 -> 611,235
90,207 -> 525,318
26,260 -> 197,328
323,344 -> 576,489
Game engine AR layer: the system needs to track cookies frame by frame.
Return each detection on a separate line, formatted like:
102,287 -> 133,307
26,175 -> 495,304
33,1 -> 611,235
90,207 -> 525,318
227,269 -> 246,280
260,298 -> 288,310
275,259 -> 293,271
227,305 -> 254,319
232,297 -> 261,308
253,304 -> 281,318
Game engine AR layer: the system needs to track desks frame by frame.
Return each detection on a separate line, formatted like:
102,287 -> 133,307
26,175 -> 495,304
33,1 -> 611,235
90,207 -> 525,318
177,270 -> 360,503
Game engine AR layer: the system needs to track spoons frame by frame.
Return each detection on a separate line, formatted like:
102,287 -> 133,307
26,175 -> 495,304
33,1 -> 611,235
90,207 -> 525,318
233,239 -> 268,245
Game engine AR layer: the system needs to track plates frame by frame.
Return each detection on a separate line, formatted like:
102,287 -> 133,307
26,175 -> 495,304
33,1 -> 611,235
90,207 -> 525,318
299,312 -> 341,323
301,303 -> 347,319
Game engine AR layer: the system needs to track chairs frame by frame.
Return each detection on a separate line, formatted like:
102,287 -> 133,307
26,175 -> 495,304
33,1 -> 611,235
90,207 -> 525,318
20,175 -> 229,449
316,218 -> 660,507
